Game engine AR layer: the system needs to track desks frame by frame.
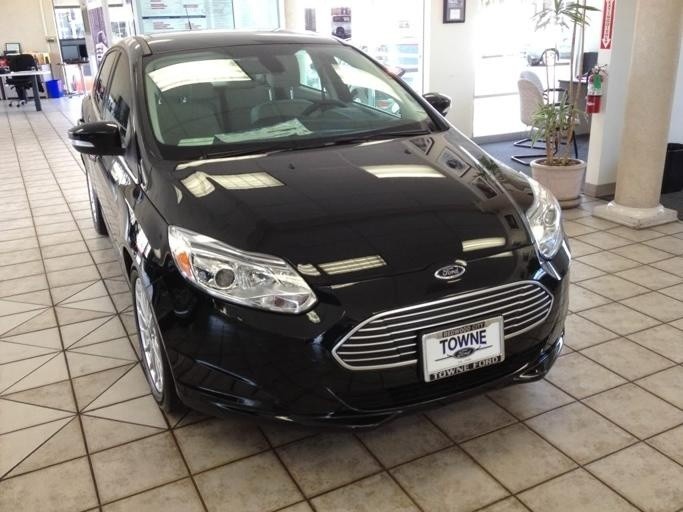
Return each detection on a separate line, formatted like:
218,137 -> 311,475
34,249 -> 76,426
556,76 -> 587,116
65,60 -> 89,97
0,70 -> 51,114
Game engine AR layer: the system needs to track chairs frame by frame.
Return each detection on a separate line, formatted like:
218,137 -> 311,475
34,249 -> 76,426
2,53 -> 43,108
510,80 -> 578,166
512,69 -> 574,149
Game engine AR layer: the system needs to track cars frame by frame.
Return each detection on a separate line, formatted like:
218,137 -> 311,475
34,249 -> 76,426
526,32 -> 572,66
63,27 -> 575,435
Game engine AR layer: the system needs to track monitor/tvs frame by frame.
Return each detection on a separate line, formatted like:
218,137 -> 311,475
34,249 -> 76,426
582,52 -> 598,77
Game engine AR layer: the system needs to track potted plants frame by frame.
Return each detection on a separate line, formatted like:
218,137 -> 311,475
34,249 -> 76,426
530,1 -> 606,210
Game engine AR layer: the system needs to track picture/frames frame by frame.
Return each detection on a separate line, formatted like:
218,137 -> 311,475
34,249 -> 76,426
4,43 -> 19,54
441,0 -> 468,24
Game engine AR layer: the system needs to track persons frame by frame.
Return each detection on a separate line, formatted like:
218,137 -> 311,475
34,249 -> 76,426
95,31 -> 108,65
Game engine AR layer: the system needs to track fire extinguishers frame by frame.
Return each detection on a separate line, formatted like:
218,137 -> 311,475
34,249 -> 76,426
585,64 -> 608,113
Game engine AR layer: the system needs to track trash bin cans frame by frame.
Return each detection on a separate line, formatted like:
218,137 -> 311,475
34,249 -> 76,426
661,143 -> 683,194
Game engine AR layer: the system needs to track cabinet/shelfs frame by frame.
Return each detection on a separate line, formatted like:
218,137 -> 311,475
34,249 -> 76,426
0,65 -> 52,99
